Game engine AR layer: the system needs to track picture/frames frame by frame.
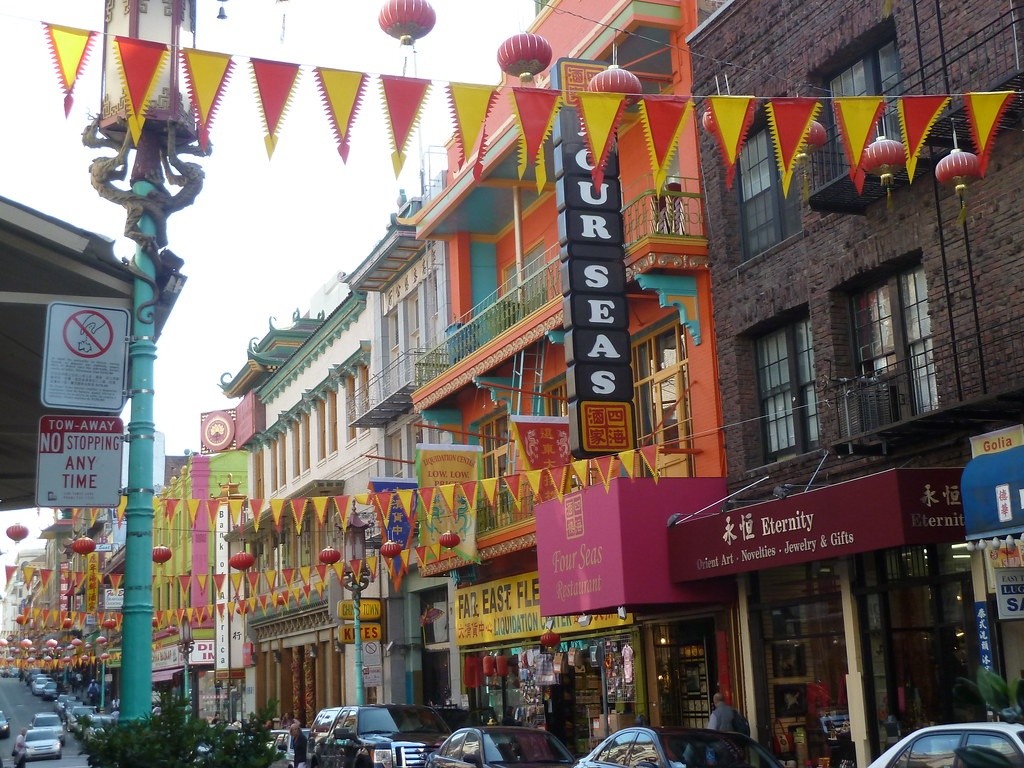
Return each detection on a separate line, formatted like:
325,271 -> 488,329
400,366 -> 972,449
771,604 -> 803,637
771,642 -> 807,678
773,683 -> 810,719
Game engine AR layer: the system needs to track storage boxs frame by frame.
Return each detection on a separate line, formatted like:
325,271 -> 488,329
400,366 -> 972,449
598,713 -> 635,739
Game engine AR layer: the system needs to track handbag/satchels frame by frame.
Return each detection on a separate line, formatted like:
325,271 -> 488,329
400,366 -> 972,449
89,694 -> 93,698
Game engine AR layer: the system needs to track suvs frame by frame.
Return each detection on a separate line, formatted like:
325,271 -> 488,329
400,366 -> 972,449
306,704 -> 452,768
29,712 -> 68,745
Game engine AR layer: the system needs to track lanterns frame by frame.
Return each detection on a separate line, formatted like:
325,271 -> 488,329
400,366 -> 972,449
380,539 -> 401,560
438,530 -> 460,550
6,523 -> 29,544
483,655 -> 509,677
229,551 -> 254,571
540,631 -> 561,653
0,615 -> 117,663
153,544 -> 172,565
319,545 -> 340,567
153,617 -> 162,627
935,148 -> 982,225
73,535 -> 95,557
378,0 -> 436,46
587,64 -> 642,108
702,107 -> 754,135
497,34 -> 552,83
795,120 -> 827,201
864,135 -> 907,212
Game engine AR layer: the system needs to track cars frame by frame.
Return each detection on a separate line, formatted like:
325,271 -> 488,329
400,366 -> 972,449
863,721 -> 1024,768
23,729 -> 63,761
83,713 -> 118,741
425,726 -> 575,768
267,729 -> 312,768
0,711 -> 10,736
572,725 -> 783,768
0,665 -> 99,736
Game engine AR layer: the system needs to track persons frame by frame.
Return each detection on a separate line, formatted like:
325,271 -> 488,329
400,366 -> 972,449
265,719 -> 274,729
87,678 -> 100,705
111,696 -> 120,712
15,727 -> 29,768
211,713 -> 219,725
281,712 -> 301,728
153,704 -> 162,716
76,672 -> 83,682
290,724 -> 308,768
707,693 -> 734,733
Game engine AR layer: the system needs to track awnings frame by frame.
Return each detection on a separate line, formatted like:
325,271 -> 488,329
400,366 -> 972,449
152,668 -> 184,681
532,439 -> 1023,617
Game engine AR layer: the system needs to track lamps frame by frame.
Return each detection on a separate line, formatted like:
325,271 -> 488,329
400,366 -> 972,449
546,616 -> 554,631
772,484 -> 828,499
578,613 -> 592,626
720,500 -> 767,512
667,513 -> 718,527
311,643 -> 318,658
617,605 -> 627,621
334,640 -> 344,653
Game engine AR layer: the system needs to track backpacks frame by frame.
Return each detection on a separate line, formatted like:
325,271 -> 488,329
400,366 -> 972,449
730,709 -> 750,736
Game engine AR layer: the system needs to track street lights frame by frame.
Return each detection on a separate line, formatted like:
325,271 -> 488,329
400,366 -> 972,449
341,498 -> 376,704
82,1 -> 213,742
177,613 -> 200,726
